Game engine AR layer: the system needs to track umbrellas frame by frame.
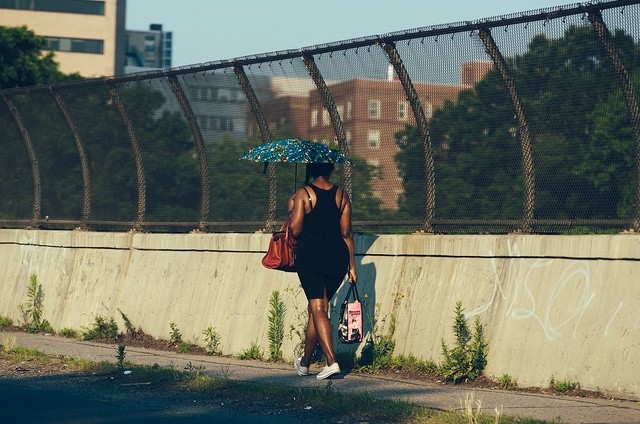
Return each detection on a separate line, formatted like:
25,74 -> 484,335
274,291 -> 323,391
239,138 -> 357,195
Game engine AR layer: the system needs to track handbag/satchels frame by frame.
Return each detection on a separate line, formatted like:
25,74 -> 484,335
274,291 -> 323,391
262,214 -> 297,272
338,279 -> 363,344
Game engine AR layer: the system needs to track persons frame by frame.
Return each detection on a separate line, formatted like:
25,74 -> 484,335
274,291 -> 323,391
288,163 -> 358,379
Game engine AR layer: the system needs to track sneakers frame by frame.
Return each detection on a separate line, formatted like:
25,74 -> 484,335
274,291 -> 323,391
316,360 -> 341,380
294,357 -> 309,376
